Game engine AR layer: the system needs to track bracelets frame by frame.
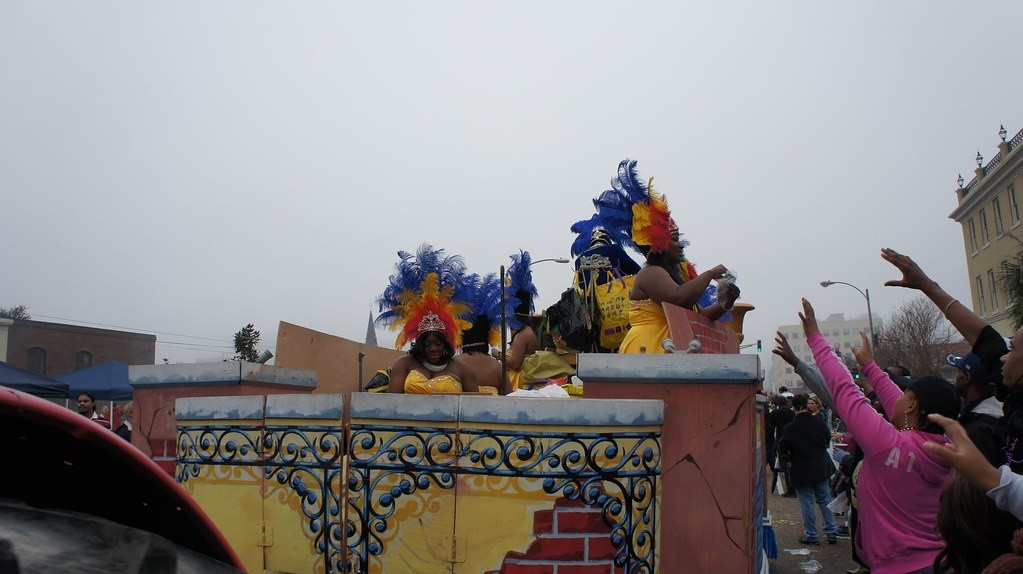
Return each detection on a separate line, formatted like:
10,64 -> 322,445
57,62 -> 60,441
945,300 -> 959,318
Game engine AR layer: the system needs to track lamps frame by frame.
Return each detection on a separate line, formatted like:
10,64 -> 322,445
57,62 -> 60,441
957,174 -> 964,188
999,124 -> 1007,142
975,151 -> 983,167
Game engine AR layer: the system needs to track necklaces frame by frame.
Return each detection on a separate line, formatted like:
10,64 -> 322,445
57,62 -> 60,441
422,360 -> 448,371
899,427 -> 914,433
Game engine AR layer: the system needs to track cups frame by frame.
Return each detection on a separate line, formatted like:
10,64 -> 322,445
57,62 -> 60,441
835,444 -> 848,452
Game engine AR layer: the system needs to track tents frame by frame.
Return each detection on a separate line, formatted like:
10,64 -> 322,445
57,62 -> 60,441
0,360 -> 70,410
54,359 -> 134,409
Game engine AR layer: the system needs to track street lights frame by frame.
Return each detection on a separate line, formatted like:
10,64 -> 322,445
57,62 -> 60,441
499,257 -> 571,396
820,280 -> 877,363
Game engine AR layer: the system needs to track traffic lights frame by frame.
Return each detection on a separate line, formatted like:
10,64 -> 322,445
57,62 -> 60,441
757,338 -> 763,354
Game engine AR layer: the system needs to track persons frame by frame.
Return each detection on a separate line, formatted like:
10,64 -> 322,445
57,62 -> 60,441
886,369 -> 913,391
778,395 -> 837,544
389,328 -> 479,395
757,386 -> 797,497
454,315 -> 514,395
115,400 -> 134,443
77,392 -> 111,429
832,445 -> 869,573
881,362 -> 910,376
101,405 -> 110,420
808,397 -> 823,417
880,247 -> 1023,471
772,299 -> 1023,574
832,429 -> 857,444
620,224 -> 740,354
493,288 -> 537,395
